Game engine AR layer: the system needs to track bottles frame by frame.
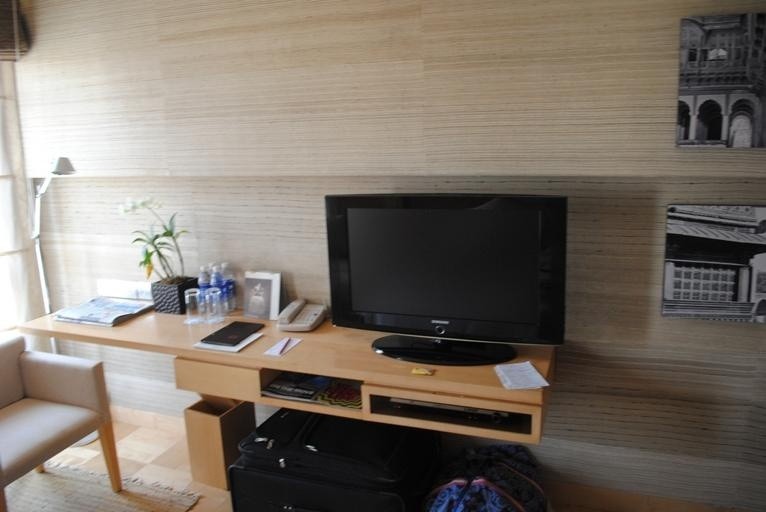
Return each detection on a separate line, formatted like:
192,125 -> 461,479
197,262 -> 238,314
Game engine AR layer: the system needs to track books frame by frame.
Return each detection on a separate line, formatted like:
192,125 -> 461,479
262,371 -> 331,400
201,319 -> 264,344
193,332 -> 264,353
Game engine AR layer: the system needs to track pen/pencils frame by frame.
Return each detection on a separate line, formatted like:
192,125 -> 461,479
279,337 -> 291,354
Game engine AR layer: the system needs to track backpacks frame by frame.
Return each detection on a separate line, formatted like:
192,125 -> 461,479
423,442 -> 546,512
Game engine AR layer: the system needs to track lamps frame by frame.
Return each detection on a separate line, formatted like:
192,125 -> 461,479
31,156 -> 103,448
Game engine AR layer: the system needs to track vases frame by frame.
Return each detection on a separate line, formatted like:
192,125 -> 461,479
149,275 -> 197,316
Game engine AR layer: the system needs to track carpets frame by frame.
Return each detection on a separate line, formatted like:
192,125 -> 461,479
1,457 -> 205,511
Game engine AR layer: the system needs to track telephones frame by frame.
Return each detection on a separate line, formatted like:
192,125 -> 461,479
276,298 -> 327,331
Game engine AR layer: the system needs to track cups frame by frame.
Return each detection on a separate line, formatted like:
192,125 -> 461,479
202,287 -> 224,324
184,288 -> 204,325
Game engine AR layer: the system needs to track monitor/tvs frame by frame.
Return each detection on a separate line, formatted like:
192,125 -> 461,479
326,194 -> 568,365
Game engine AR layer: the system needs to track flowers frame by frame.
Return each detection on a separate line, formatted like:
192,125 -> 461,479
118,190 -> 184,283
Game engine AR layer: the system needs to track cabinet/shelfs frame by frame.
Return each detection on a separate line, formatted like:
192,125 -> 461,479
225,458 -> 407,512
18,296 -> 558,448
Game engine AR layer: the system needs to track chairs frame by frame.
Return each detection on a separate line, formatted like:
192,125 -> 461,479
0,336 -> 124,511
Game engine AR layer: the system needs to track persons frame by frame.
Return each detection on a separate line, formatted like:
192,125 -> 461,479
247,281 -> 267,316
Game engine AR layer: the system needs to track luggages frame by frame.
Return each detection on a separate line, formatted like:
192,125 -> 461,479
226,407 -> 444,512
